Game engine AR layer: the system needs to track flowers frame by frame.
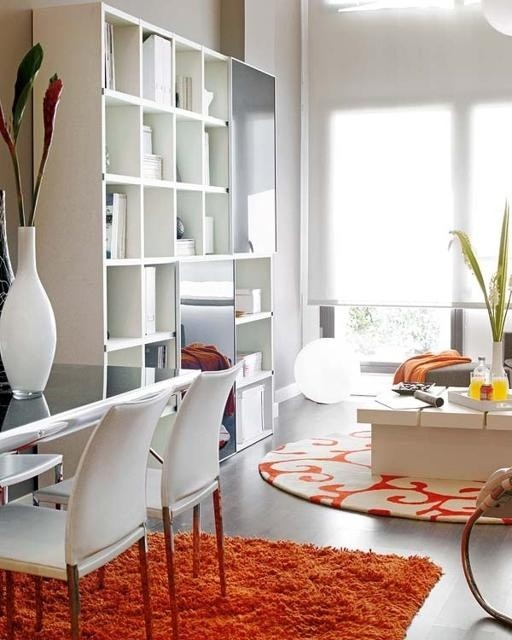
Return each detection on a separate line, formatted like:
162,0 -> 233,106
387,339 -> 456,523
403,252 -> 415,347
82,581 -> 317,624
0,43 -> 64,226
446,197 -> 512,342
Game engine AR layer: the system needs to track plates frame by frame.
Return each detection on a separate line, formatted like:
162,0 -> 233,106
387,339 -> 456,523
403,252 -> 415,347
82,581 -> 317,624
391,381 -> 436,395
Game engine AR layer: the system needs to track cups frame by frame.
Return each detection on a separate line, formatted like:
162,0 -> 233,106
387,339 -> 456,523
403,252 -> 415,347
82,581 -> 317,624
470,372 -> 486,400
492,373 -> 508,400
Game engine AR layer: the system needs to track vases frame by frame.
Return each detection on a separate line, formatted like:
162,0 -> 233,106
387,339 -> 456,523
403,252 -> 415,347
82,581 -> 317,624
0,226 -> 57,401
490,342 -> 509,392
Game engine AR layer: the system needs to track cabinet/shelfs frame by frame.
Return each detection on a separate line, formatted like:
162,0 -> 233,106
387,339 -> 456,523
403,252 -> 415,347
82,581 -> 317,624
30,1 -> 277,491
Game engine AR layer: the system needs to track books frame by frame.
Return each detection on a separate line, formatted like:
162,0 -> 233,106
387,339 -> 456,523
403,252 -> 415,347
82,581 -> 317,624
142,154 -> 163,179
104,23 -> 116,91
142,32 -> 172,107
177,76 -> 193,111
105,192 -> 127,259
237,349 -> 262,378
177,237 -> 197,257
143,267 -> 156,337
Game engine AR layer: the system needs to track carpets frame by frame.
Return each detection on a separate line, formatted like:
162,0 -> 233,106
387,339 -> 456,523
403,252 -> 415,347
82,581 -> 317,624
0,530 -> 442,640
258,428 -> 512,525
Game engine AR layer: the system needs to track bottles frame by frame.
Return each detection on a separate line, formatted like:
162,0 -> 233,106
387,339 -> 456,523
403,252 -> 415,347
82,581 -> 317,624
473,355 -> 490,384
